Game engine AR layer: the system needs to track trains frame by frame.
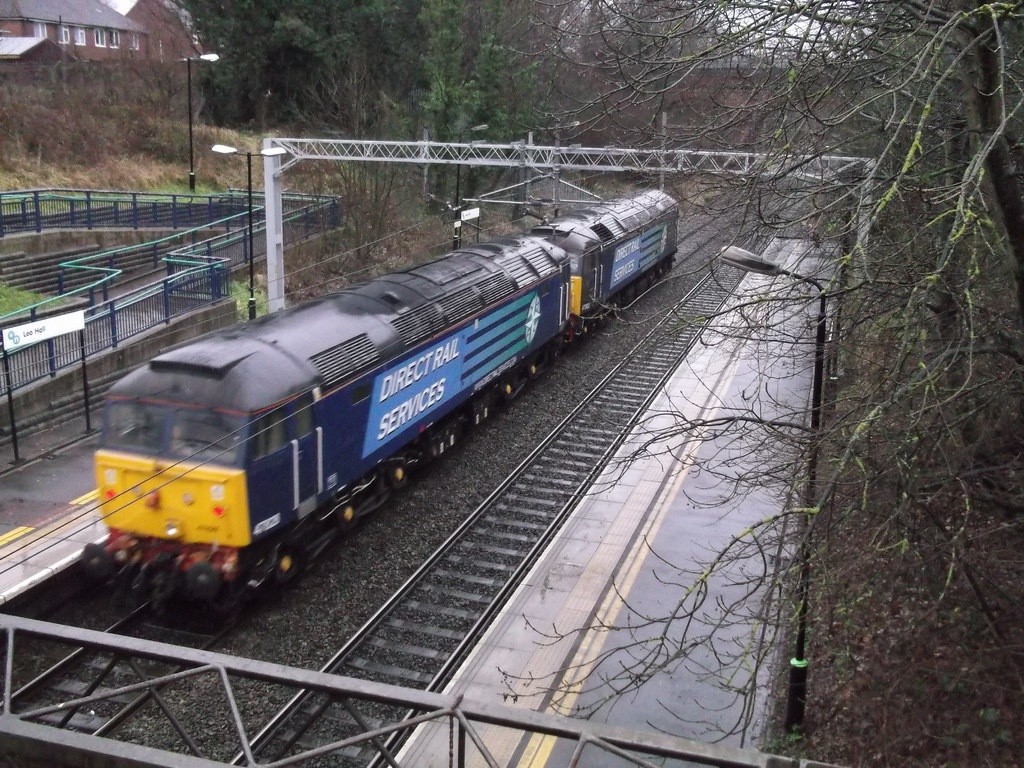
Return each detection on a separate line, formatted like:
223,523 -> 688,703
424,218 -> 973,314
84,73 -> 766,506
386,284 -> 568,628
79,188 -> 681,624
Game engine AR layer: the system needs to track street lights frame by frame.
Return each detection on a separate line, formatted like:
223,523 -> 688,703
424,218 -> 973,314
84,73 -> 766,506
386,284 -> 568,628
210,143 -> 289,322
174,51 -> 220,192
715,244 -> 827,531
454,124 -> 489,250
